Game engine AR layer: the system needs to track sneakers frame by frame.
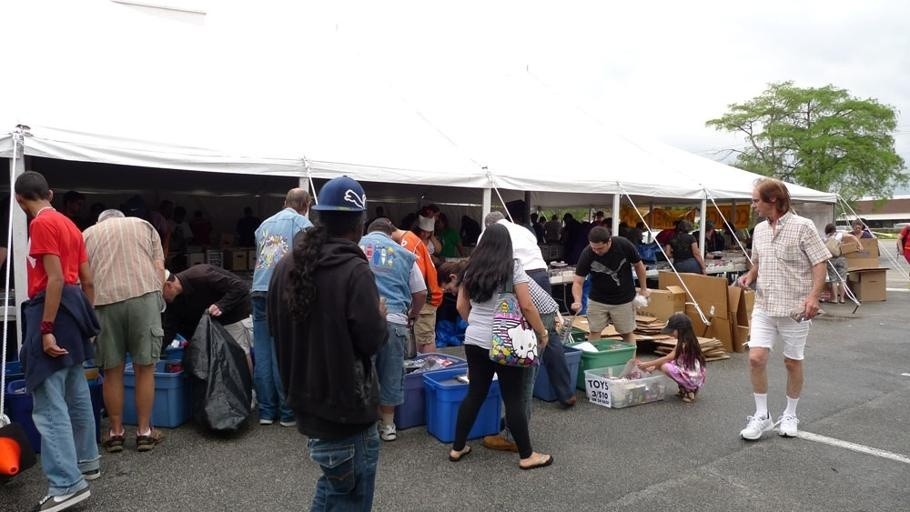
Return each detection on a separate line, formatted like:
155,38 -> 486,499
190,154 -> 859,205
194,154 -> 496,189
280,417 -> 296,428
81,470 -> 101,481
29,486 -> 90,511
257,417 -> 273,425
99,425 -> 128,452
135,424 -> 166,451
739,411 -> 775,440
379,422 -> 398,442
779,412 -> 799,437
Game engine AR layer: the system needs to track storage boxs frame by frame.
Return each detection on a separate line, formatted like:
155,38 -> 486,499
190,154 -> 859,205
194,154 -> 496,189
534,347 -> 583,402
187,252 -> 205,267
225,248 -> 248,272
422,367 -> 502,443
583,364 -> 666,408
120,359 -> 196,429
636,288 -> 686,314
2,358 -> 26,376
6,376 -> 102,445
850,266 -> 889,301
386,353 -> 469,430
249,250 -> 257,272
841,243 -> 860,254
163,336 -> 190,360
207,250 -> 224,269
568,338 -> 636,390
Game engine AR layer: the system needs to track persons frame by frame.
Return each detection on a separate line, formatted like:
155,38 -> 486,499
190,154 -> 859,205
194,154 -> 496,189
385,223 -> 443,354
428,204 -> 440,221
448,223 -> 555,468
81,208 -> 166,456
476,212 -> 578,407
172,206 -> 193,252
356,218 -> 429,442
723,220 -> 743,249
159,200 -> 176,232
266,174 -> 388,511
437,255 -> 558,453
594,211 -> 603,225
414,208 -> 442,264
572,227 -> 652,356
251,188 -> 314,428
0,203 -> 8,269
544,214 -> 563,242
163,263 -> 256,409
736,178 -> 832,444
539,216 -> 546,229
897,225 -> 910,264
190,211 -> 210,243
602,217 -> 611,234
123,196 -> 168,258
58,191 -> 83,229
562,213 -> 581,264
705,224 -> 722,259
656,221 -> 679,245
846,220 -> 872,239
637,314 -> 706,403
530,213 -> 542,244
820,224 -> 863,304
435,212 -> 465,258
13,170 -> 102,512
236,207 -> 254,246
666,220 -> 707,277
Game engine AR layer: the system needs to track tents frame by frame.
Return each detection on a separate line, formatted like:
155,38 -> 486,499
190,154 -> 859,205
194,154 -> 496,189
0,66 -> 842,355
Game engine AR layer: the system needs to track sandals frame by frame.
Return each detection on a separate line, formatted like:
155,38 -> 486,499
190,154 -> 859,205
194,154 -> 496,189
675,385 -> 686,398
683,387 -> 700,403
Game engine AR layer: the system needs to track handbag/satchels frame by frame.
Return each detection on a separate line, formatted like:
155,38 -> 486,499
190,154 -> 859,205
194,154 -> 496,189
488,258 -> 540,368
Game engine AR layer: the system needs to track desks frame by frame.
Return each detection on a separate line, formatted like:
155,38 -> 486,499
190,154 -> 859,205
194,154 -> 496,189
703,250 -> 753,282
631,256 -> 671,288
545,263 -> 590,312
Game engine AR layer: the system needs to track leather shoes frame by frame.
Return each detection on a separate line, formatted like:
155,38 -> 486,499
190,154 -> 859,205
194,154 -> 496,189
564,396 -> 576,408
483,434 -> 517,450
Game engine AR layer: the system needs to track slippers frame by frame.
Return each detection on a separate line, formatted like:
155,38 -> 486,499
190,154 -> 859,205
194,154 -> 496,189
519,453 -> 553,470
448,441 -> 472,462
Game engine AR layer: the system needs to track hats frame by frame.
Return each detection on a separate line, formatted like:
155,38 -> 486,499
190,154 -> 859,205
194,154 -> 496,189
660,314 -> 693,332
419,215 -> 435,232
309,177 -> 368,212
